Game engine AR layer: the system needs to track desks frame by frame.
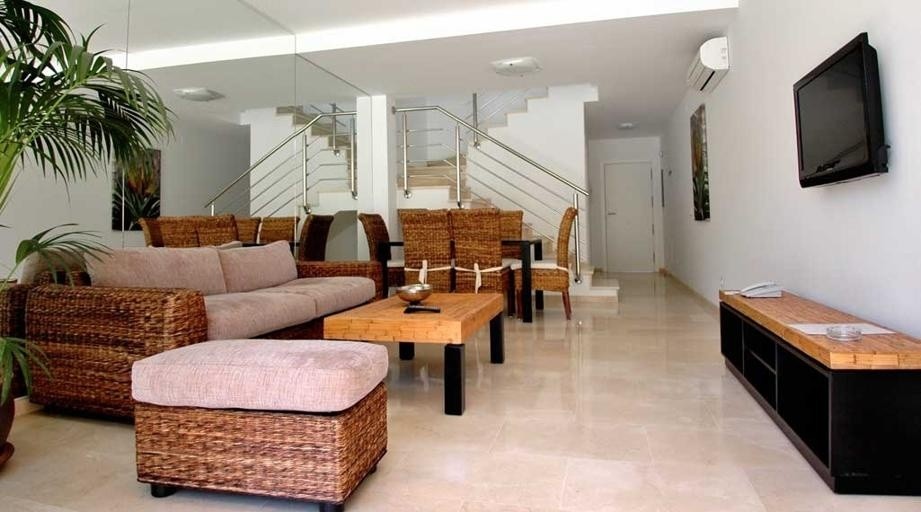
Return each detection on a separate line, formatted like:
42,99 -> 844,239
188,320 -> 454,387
376,238 -> 545,322
242,242 -> 300,257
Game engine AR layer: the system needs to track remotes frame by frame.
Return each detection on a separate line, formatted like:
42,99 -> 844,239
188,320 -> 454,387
404,305 -> 440,313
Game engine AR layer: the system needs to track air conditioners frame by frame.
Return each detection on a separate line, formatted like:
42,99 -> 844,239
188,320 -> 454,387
686,36 -> 731,97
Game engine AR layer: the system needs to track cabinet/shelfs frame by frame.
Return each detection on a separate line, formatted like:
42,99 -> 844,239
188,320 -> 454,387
719,289 -> 921,496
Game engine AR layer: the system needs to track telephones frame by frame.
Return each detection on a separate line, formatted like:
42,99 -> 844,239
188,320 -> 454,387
723,280 -> 783,298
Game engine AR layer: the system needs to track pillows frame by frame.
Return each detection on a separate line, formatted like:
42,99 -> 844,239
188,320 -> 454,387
218,239 -> 299,293
17,247 -> 87,285
83,245 -> 228,296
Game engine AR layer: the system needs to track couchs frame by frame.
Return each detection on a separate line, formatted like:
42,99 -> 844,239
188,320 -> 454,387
131,339 -> 390,512
25,261 -> 384,419
0,267 -> 86,401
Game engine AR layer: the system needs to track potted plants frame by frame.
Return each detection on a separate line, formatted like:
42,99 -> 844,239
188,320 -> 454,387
0,0 -> 182,474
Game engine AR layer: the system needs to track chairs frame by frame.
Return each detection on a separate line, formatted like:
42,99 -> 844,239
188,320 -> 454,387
260,216 -> 301,241
138,217 -> 165,247
358,213 -> 405,299
192,213 -> 240,247
451,208 -> 514,317
510,207 -> 578,320
499,211 -> 524,316
234,216 -> 262,243
298,214 -> 335,261
398,209 -> 454,293
157,215 -> 200,248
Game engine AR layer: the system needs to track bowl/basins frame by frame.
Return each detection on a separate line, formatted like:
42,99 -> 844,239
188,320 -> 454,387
396,284 -> 433,302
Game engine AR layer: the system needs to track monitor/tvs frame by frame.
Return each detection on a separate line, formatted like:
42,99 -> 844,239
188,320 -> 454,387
792,32 -> 888,188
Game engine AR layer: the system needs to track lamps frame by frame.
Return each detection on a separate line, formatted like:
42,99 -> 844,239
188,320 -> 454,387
172,86 -> 226,102
616,120 -> 641,132
491,56 -> 546,78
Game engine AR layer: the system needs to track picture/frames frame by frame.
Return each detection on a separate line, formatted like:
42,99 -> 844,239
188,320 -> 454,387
690,103 -> 711,222
112,146 -> 162,231
